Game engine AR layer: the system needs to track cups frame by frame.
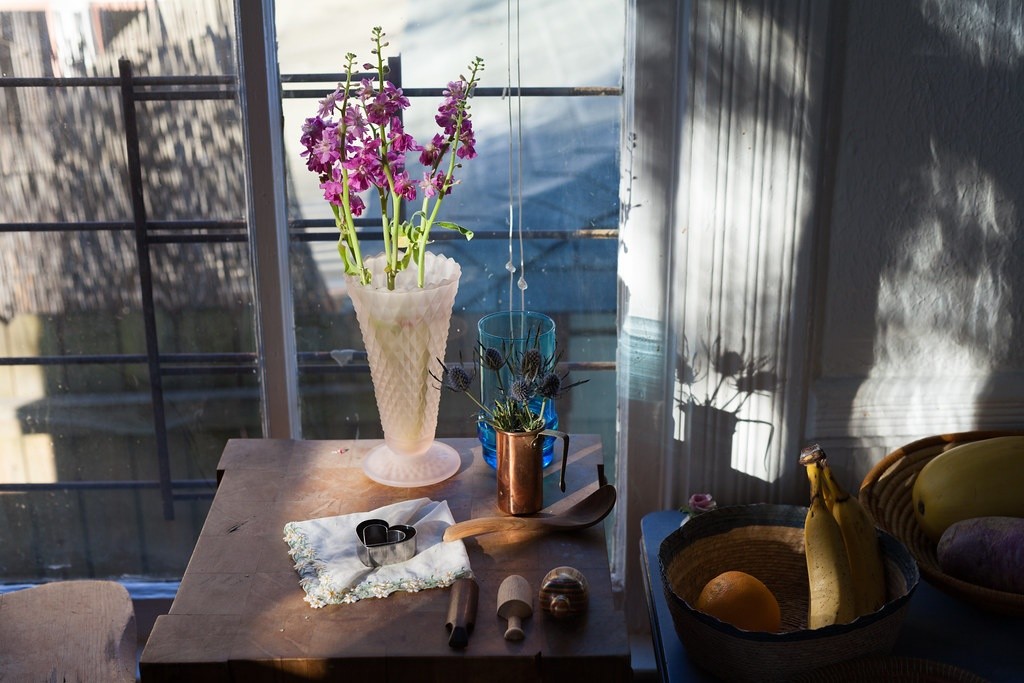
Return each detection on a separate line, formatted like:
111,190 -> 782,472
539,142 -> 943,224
478,311 -> 559,470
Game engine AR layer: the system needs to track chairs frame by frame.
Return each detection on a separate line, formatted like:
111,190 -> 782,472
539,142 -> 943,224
1,574 -> 141,683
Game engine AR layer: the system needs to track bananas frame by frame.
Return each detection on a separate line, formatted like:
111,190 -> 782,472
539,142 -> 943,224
797,443 -> 885,631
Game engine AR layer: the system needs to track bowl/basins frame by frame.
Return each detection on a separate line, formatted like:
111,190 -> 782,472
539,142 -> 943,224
860,429 -> 1024,624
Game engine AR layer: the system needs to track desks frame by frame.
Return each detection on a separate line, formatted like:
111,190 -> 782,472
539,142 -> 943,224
137,427 -> 631,683
640,506 -> 1024,683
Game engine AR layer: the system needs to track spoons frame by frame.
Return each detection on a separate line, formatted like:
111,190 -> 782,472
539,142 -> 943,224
442,484 -> 618,544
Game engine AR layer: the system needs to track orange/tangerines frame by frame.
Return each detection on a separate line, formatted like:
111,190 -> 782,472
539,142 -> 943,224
697,571 -> 783,633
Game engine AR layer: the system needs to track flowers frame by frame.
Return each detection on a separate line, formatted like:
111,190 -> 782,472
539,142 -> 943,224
426,319 -> 594,432
678,490 -> 716,520
300,25 -> 486,289
673,329 -> 786,471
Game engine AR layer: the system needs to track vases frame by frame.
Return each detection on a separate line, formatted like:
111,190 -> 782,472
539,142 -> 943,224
495,426 -> 571,516
676,402 -> 736,500
341,252 -> 462,489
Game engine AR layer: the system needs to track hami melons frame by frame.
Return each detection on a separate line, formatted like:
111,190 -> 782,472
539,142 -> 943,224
912,436 -> 1023,547
